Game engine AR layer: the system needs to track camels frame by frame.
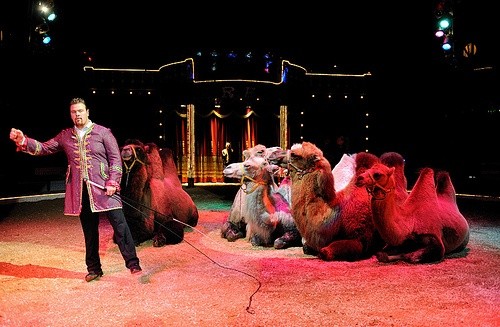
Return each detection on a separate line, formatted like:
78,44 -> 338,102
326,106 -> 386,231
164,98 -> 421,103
218,143 -> 301,250
354,163 -> 472,268
263,141 -> 409,266
114,141 -> 200,249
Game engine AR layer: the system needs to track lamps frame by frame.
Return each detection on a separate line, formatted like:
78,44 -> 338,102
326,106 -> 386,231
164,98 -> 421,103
442,34 -> 452,51
43,34 -> 52,45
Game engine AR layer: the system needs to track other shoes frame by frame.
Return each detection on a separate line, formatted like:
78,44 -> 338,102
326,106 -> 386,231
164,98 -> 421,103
130,265 -> 142,274
86,272 -> 102,282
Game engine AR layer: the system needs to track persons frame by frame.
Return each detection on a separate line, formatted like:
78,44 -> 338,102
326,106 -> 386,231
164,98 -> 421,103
10,98 -> 142,282
222,143 -> 234,183
